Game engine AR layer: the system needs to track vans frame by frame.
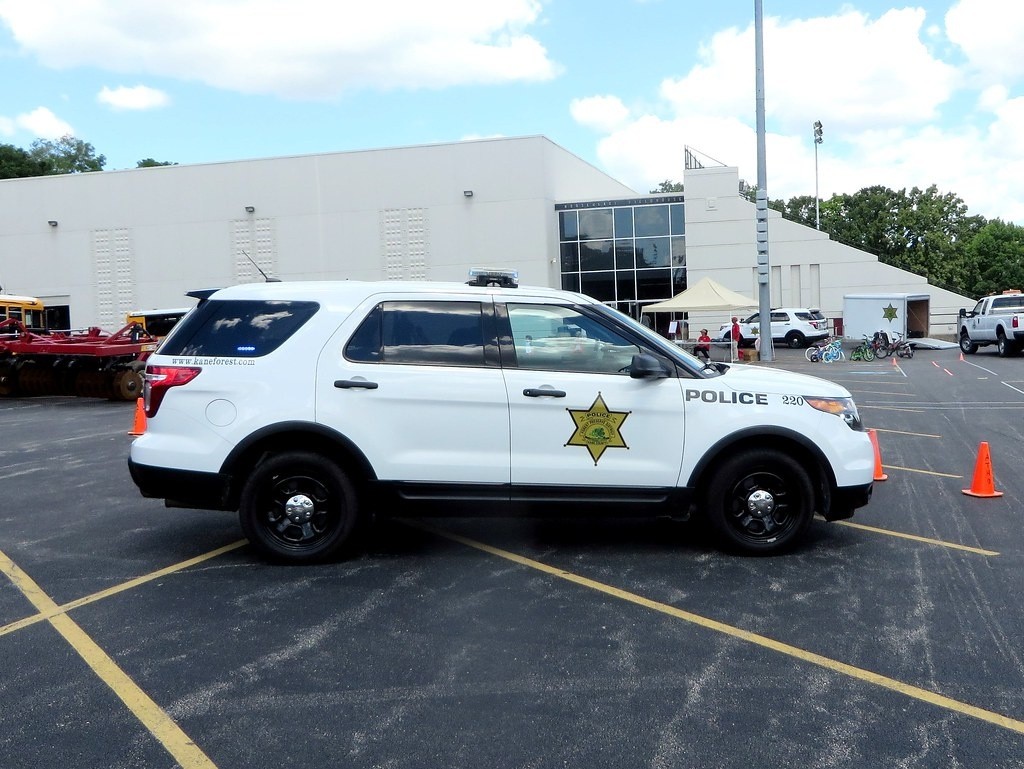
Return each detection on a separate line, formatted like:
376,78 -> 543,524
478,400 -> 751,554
127,308 -> 191,349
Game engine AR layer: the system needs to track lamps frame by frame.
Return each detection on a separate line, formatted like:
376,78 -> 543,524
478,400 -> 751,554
464,191 -> 473,197
48,221 -> 57,226
245,207 -> 254,212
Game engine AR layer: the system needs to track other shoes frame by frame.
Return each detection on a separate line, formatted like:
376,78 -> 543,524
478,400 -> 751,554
707,357 -> 711,362
733,357 -> 739,361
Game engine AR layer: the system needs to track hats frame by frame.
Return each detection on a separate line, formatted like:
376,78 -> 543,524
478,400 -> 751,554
731,316 -> 738,320
700,328 -> 708,332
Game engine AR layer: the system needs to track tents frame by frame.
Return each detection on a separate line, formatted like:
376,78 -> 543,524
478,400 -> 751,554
638,277 -> 776,363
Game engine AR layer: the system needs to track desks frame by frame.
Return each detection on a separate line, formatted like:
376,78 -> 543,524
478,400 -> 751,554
675,342 -> 735,363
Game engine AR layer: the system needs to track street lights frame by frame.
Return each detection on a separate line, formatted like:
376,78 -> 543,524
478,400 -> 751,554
813,119 -> 823,230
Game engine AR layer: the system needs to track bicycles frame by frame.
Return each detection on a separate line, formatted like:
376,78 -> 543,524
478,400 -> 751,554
851,330 -> 913,361
805,336 -> 845,363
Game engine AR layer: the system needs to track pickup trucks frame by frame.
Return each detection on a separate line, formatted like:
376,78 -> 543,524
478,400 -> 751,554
959,293 -> 1024,355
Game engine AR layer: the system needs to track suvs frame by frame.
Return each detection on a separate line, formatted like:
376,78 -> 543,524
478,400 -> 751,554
720,308 -> 830,348
129,249 -> 875,560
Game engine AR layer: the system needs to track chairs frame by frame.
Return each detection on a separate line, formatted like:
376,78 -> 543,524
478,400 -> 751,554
684,338 -> 711,361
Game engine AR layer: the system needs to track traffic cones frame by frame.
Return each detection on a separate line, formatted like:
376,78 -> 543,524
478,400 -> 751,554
959,353 -> 964,360
869,429 -> 887,480
892,358 -> 896,366
961,442 -> 1003,497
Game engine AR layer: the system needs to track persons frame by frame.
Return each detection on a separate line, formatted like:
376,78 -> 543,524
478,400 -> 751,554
694,329 -> 712,362
730,316 -> 740,361
